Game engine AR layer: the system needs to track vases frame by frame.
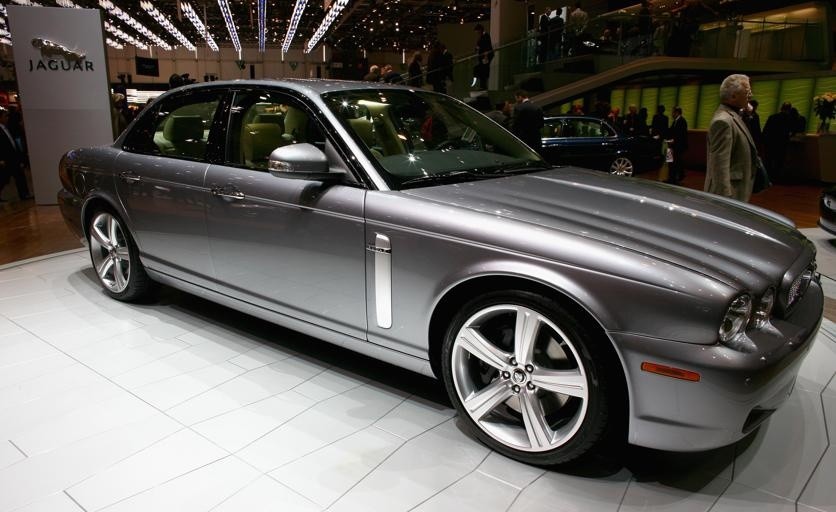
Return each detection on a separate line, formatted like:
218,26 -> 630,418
816,116 -> 830,135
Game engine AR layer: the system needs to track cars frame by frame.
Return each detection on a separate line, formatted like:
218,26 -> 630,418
541,114 -> 663,179
561,33 -> 624,57
57,78 -> 825,468
818,181 -> 836,236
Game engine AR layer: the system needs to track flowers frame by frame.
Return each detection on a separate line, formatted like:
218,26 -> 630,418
814,92 -> 836,129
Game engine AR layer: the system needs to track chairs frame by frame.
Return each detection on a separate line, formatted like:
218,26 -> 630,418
253,113 -> 286,135
240,124 -> 284,170
345,117 -> 384,162
162,115 -> 206,161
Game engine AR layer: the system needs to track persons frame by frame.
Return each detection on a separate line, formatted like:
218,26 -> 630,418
0,1 -> 809,202
